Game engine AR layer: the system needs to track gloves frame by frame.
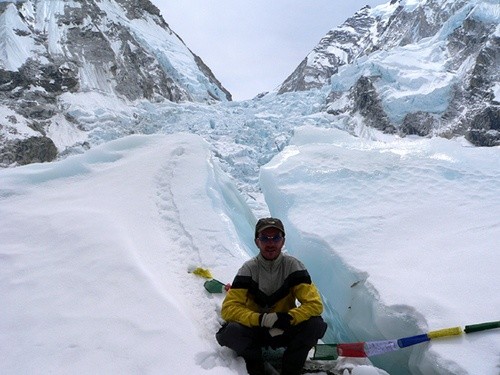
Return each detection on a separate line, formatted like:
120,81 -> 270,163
264,327 -> 284,350
261,312 -> 294,332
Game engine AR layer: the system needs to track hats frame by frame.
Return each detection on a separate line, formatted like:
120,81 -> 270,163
255,217 -> 285,235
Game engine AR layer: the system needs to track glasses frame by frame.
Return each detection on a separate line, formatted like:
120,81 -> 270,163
256,235 -> 284,242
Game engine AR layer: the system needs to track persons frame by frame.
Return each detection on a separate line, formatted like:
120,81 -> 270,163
216,217 -> 330,374
